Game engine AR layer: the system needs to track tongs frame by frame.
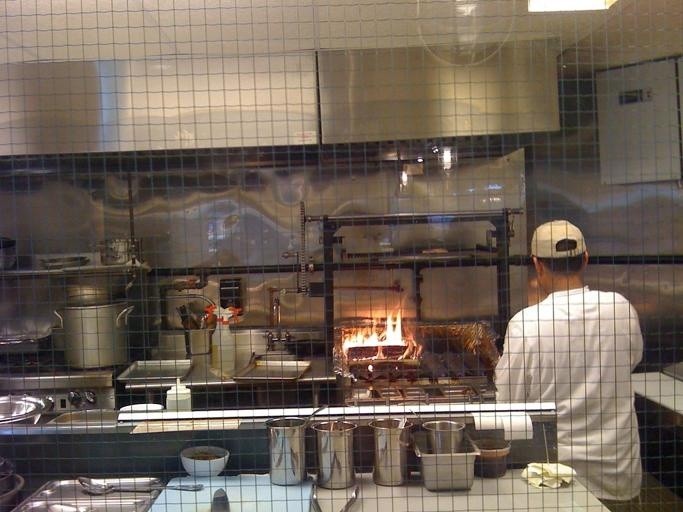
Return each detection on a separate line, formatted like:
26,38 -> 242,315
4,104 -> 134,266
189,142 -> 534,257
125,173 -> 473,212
310,486 -> 360,511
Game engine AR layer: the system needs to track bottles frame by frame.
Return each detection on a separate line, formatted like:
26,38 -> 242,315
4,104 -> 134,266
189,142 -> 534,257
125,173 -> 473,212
272,296 -> 281,327
212,317 -> 222,368
217,320 -> 235,373
166,379 -> 191,409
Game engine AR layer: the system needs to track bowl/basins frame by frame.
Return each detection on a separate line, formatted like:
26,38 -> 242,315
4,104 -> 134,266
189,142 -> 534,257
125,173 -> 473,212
472,440 -> 512,479
0,395 -> 52,424
180,446 -> 230,476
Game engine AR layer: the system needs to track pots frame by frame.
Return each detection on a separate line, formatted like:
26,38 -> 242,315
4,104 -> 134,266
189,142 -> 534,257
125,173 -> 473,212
52,302 -> 134,368
90,238 -> 143,264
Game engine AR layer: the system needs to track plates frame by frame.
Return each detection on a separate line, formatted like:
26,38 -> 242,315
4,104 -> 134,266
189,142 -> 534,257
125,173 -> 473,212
38,256 -> 90,268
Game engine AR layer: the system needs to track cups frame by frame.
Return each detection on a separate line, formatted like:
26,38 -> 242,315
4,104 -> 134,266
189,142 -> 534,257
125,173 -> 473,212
421,420 -> 465,453
266,417 -> 307,485
311,422 -> 357,488
368,417 -> 412,487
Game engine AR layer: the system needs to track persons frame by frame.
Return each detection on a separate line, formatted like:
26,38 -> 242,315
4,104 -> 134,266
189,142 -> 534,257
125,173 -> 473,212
494,219 -> 645,511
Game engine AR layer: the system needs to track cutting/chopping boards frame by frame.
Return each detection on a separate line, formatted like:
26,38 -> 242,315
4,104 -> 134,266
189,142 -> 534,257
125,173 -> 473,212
147,475 -> 312,512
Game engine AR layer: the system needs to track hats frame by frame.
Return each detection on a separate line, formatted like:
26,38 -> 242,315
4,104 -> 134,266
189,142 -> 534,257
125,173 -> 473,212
529,219 -> 586,259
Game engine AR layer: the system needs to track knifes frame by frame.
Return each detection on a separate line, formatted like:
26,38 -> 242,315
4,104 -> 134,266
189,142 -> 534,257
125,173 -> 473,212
211,488 -> 229,511
